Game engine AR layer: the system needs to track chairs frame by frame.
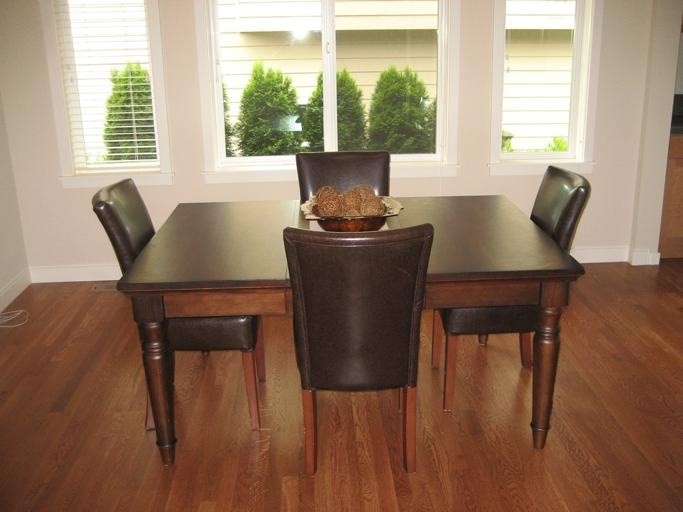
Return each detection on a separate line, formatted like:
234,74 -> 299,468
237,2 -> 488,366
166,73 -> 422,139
431,166 -> 591,418
296,150 -> 391,200
282,224 -> 434,476
91,176 -> 267,432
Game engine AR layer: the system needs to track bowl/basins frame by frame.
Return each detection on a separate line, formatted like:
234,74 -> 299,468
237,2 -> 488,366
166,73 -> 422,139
299,197 -> 405,234
